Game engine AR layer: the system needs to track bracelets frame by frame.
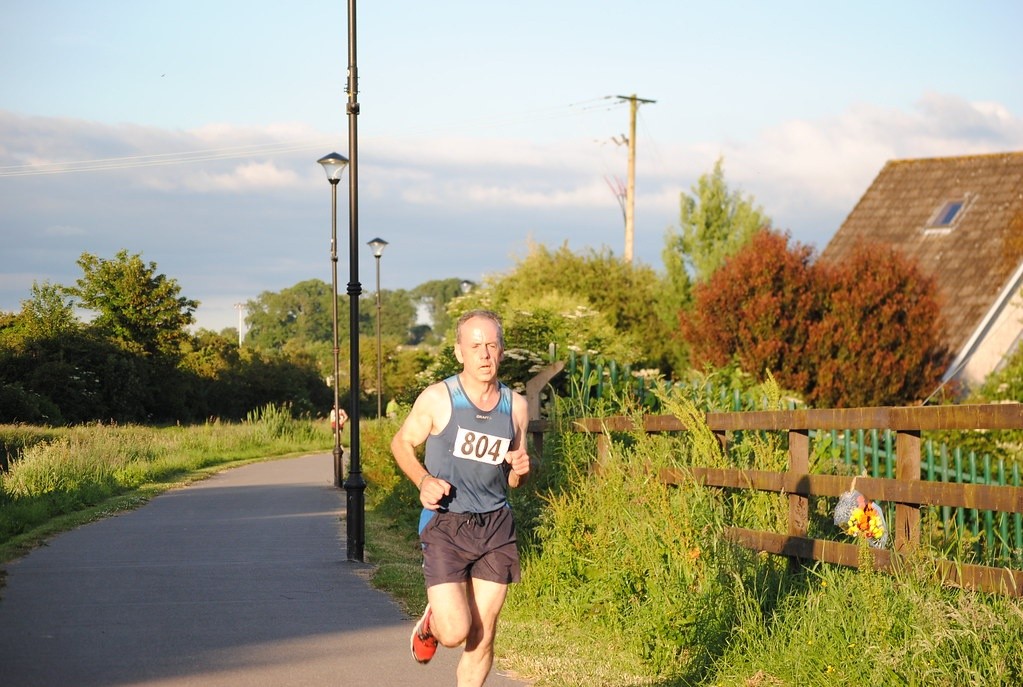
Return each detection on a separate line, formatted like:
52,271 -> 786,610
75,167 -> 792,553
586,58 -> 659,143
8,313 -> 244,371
418,474 -> 432,491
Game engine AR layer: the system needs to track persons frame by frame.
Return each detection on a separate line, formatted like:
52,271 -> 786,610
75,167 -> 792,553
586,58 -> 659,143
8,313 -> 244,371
331,403 -> 348,450
390,311 -> 529,687
386,398 -> 400,418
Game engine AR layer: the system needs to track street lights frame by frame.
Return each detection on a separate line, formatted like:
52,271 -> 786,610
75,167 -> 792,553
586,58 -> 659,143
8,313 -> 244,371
317,150 -> 350,489
365,237 -> 389,430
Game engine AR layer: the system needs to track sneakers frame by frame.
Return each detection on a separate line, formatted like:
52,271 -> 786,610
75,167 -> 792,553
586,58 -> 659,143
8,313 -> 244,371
410,603 -> 438,664
